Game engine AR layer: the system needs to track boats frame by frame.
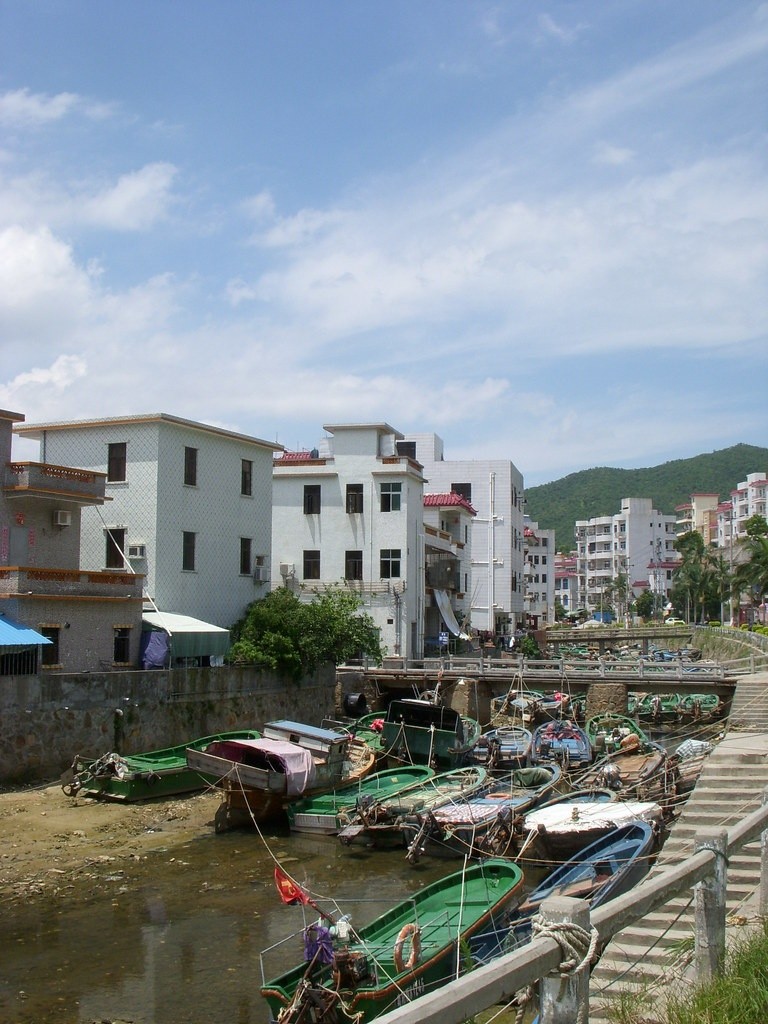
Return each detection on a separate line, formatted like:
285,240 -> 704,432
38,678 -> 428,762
259,857 -> 526,1024
464,820 -> 653,974
55,643 -> 732,864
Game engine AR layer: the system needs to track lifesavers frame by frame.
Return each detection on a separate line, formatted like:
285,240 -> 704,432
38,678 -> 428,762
485,793 -> 512,801
392,922 -> 421,973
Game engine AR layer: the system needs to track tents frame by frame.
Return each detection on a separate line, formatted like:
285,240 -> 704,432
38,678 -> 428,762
142,611 -> 230,670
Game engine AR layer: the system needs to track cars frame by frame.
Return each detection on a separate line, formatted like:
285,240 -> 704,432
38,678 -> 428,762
664,617 -> 686,625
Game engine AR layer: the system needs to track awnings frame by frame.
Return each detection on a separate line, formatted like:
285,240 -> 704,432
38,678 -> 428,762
0,615 -> 53,645
